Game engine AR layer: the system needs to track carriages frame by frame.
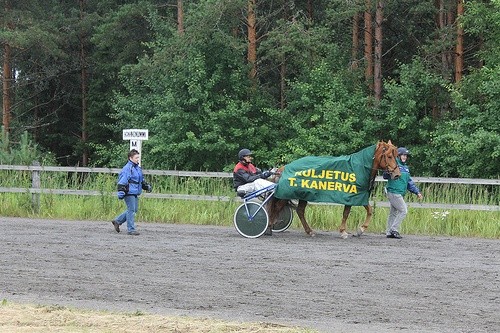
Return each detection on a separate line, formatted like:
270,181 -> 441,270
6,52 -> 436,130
233,140 -> 401,241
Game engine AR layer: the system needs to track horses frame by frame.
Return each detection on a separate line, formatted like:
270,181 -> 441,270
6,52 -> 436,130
267,138 -> 402,240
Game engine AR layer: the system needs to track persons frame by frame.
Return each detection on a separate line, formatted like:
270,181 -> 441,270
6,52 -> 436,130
112,149 -> 152,235
233,148 -> 278,197
383,147 -> 422,239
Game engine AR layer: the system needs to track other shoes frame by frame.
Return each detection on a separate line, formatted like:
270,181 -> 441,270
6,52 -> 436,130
390,230 -> 401,239
112,220 -> 120,233
127,231 -> 140,235
387,235 -> 395,238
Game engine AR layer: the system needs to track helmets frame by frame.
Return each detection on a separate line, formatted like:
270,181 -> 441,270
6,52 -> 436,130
238,149 -> 254,158
398,148 -> 409,156
